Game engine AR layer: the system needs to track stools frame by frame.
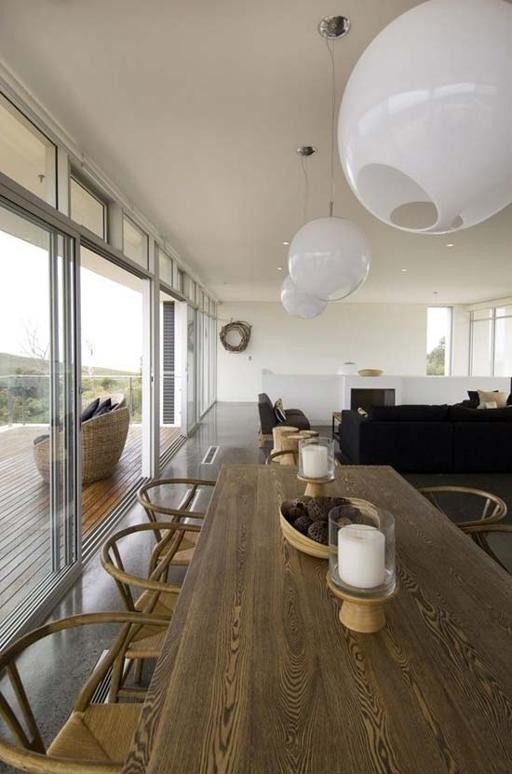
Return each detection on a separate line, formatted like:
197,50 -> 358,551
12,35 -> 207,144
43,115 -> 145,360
271,425 -> 319,464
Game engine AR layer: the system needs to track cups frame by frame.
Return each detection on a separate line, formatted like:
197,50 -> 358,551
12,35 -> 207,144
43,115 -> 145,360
328,504 -> 396,600
298,436 -> 335,482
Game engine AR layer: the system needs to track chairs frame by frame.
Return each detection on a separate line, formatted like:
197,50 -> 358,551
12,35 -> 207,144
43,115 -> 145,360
33,406 -> 130,486
47,393 -> 127,430
462,524 -> 512,575
100,522 -> 204,704
0,611 -> 171,774
417,485 -> 508,525
137,478 -> 216,581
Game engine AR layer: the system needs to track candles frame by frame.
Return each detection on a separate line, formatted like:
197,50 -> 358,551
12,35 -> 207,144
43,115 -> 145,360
302,445 -> 328,478
337,523 -> 387,589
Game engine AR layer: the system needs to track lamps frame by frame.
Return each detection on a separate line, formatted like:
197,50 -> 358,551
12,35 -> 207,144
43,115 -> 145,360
281,144 -> 328,319
289,18 -> 371,302
337,0 -> 512,237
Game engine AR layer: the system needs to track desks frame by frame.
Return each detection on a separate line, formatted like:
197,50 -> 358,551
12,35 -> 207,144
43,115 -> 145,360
125,465 -> 512,774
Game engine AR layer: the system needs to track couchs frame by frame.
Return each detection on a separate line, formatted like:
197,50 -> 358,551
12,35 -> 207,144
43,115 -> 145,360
258,393 -> 310,448
339,408 -> 512,475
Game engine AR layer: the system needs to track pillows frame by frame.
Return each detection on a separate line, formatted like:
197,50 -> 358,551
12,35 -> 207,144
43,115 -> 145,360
81,398 -> 118,423
274,399 -> 286,416
274,407 -> 287,422
467,389 -> 510,409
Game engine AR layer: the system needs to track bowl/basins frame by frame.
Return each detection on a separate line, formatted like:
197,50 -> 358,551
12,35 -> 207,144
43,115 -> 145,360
358,369 -> 383,376
